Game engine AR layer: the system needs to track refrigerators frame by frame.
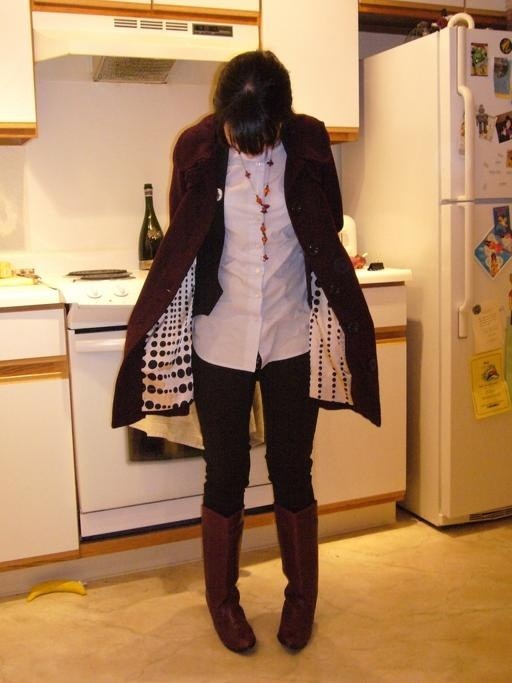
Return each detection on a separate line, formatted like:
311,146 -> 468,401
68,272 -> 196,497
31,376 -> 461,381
360,24 -> 512,527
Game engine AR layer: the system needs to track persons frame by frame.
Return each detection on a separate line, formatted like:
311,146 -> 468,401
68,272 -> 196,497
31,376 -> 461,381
111,49 -> 383,654
496,115 -> 512,142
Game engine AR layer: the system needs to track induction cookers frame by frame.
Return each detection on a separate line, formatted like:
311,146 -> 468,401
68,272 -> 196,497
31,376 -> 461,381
43,267 -> 152,330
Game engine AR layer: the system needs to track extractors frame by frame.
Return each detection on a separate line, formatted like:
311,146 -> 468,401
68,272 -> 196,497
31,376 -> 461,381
33,12 -> 259,87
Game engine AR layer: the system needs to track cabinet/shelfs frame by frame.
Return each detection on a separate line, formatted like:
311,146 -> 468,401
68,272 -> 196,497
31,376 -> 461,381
0,0 -> 38,148
0,303 -> 80,602
310,282 -> 409,541
258,2 -> 359,146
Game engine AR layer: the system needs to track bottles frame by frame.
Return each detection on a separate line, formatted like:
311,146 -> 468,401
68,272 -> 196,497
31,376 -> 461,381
139,183 -> 164,270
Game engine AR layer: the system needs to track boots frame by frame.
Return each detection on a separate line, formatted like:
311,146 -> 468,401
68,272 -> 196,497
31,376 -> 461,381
200,503 -> 255,653
272,500 -> 318,651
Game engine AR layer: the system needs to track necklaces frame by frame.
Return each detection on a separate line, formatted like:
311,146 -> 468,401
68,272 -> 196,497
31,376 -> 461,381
237,146 -> 275,260
